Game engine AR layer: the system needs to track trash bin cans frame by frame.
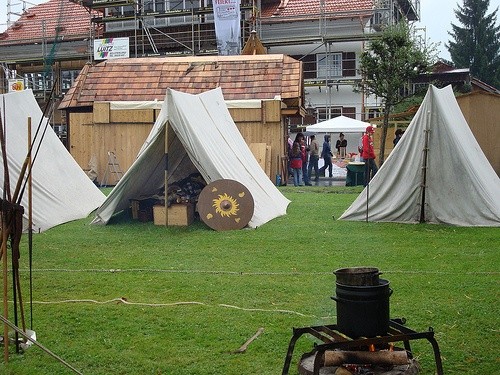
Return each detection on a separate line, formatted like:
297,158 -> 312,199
346,164 -> 366,185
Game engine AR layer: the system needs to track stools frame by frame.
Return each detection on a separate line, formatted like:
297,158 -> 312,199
128,195 -> 159,221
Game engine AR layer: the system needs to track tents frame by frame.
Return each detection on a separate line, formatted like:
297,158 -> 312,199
89,87 -> 290,230
338,83 -> 499,227
1,88 -> 107,234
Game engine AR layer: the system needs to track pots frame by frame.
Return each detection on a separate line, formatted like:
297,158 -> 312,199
330,267 -> 393,338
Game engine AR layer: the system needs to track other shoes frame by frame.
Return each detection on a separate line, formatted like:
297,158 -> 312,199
294,169 -> 333,186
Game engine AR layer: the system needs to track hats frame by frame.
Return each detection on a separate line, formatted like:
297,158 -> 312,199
366,126 -> 375,133
394,129 -> 405,134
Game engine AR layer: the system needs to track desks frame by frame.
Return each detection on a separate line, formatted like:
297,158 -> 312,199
346,161 -> 367,187
318,157 -> 358,179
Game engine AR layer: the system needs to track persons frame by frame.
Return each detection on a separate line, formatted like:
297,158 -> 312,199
335,133 -> 347,155
319,135 -> 333,177
290,133 -> 305,186
392,129 -> 405,147
306,135 -> 319,181
299,137 -> 312,186
360,126 -> 378,187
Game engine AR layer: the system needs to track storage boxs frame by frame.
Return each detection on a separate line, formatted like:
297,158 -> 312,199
152,202 -> 194,228
